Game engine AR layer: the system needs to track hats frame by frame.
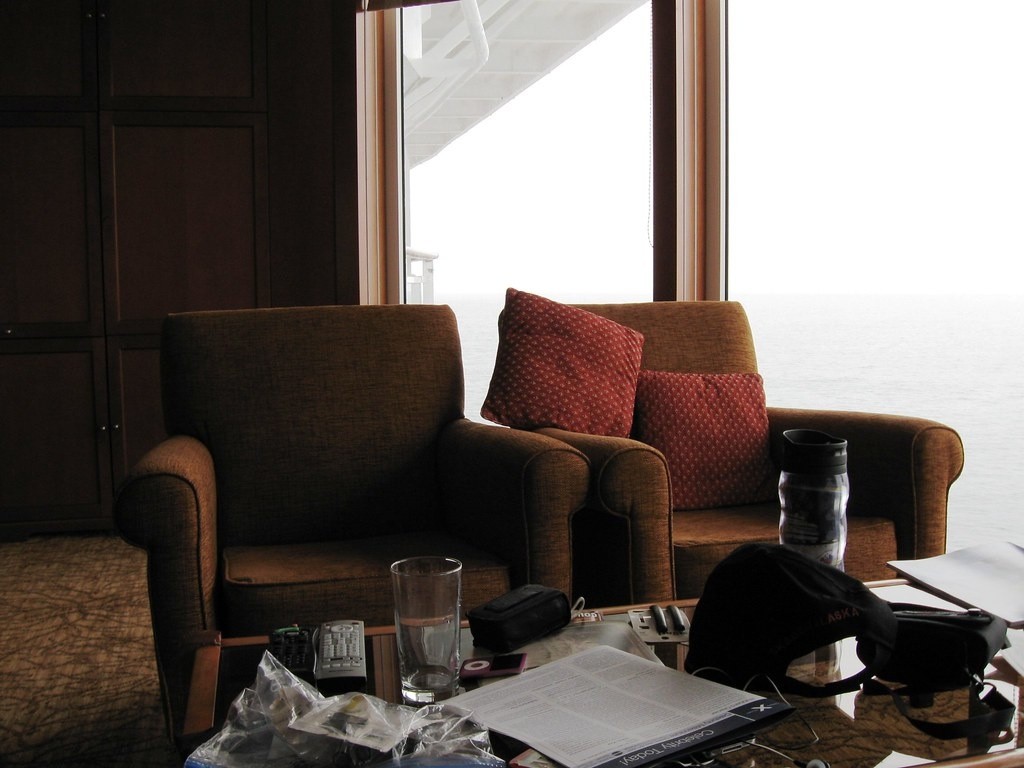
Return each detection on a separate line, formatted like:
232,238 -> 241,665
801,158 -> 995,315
684,543 -> 897,699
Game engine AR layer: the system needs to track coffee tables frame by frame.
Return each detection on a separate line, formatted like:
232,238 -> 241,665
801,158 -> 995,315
176,577 -> 1024,768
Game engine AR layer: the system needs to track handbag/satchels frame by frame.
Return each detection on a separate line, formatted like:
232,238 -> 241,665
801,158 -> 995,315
465,584 -> 586,654
856,608 -> 1016,740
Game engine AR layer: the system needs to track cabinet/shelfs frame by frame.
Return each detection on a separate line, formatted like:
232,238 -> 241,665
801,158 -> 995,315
0,0 -> 274,538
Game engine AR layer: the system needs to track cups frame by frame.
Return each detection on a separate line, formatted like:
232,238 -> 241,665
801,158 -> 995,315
778,428 -> 850,574
390,555 -> 462,704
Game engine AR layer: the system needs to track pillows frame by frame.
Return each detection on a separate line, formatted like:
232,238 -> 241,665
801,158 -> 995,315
475,287 -> 649,437
636,367 -> 773,509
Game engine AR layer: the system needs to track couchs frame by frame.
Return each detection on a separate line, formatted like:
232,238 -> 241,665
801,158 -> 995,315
129,302 -> 596,744
508,302 -> 969,600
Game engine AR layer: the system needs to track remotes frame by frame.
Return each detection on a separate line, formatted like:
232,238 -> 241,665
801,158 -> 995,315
270,623 -> 319,686
316,620 -> 367,687
